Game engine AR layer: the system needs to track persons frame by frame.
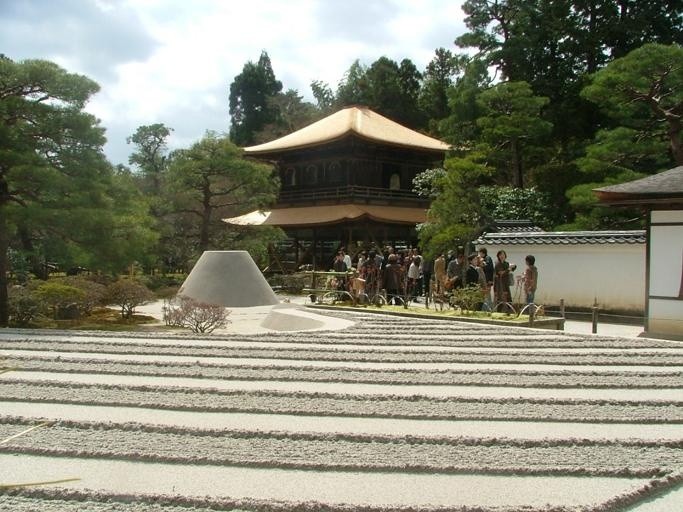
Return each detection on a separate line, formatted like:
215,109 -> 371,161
333,245 -> 538,314
